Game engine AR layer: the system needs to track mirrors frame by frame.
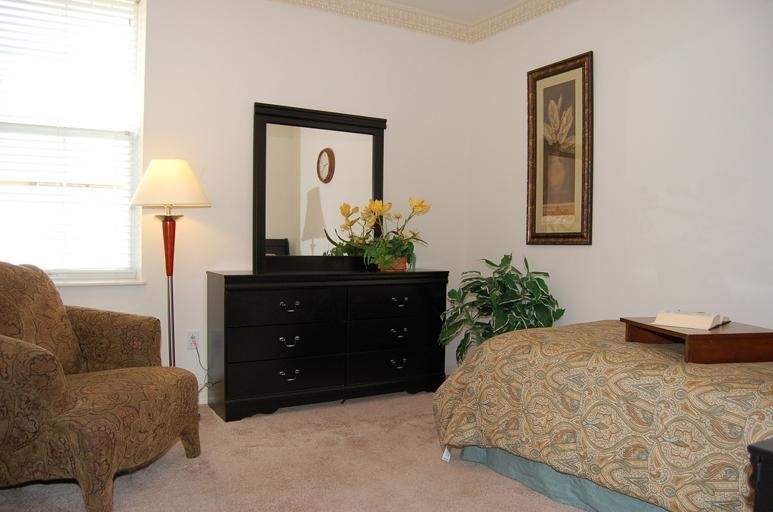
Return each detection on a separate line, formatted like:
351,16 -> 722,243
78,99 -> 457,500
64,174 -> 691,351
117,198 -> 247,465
252,101 -> 387,273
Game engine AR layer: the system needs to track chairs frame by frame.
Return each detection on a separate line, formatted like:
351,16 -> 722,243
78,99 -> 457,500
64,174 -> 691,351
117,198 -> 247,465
0,261 -> 201,512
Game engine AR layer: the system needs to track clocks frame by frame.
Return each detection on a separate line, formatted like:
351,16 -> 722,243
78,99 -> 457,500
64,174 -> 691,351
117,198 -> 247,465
316,148 -> 335,183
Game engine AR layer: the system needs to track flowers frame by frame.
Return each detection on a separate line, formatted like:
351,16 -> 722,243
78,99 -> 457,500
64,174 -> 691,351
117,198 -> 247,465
321,202 -> 364,257
334,198 -> 431,271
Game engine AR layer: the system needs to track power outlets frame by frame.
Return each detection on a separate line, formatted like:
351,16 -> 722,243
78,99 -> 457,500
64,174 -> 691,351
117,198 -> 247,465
185,331 -> 199,352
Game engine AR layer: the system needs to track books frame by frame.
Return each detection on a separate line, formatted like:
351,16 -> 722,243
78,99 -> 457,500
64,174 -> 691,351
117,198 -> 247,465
650,308 -> 732,330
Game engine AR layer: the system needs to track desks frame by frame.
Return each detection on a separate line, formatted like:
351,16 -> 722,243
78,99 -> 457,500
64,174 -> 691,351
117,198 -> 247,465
621,314 -> 773,362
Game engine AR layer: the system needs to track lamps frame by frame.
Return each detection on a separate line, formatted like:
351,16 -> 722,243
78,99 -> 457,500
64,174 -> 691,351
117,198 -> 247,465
128,158 -> 211,366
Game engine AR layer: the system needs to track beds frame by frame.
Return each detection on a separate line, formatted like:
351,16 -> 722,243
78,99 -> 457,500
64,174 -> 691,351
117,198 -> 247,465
436,318 -> 773,512
265,238 -> 290,255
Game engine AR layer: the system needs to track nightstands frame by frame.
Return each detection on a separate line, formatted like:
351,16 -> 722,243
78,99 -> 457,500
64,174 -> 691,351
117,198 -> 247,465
747,437 -> 773,512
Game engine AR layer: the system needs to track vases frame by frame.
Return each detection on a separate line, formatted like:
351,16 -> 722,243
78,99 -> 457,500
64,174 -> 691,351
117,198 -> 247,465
377,256 -> 408,272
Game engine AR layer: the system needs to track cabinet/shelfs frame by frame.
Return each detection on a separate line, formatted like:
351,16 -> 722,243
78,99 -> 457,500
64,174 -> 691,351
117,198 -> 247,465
204,270 -> 449,422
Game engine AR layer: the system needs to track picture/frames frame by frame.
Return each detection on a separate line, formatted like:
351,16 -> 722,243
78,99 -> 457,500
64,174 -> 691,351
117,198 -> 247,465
524,50 -> 594,243
